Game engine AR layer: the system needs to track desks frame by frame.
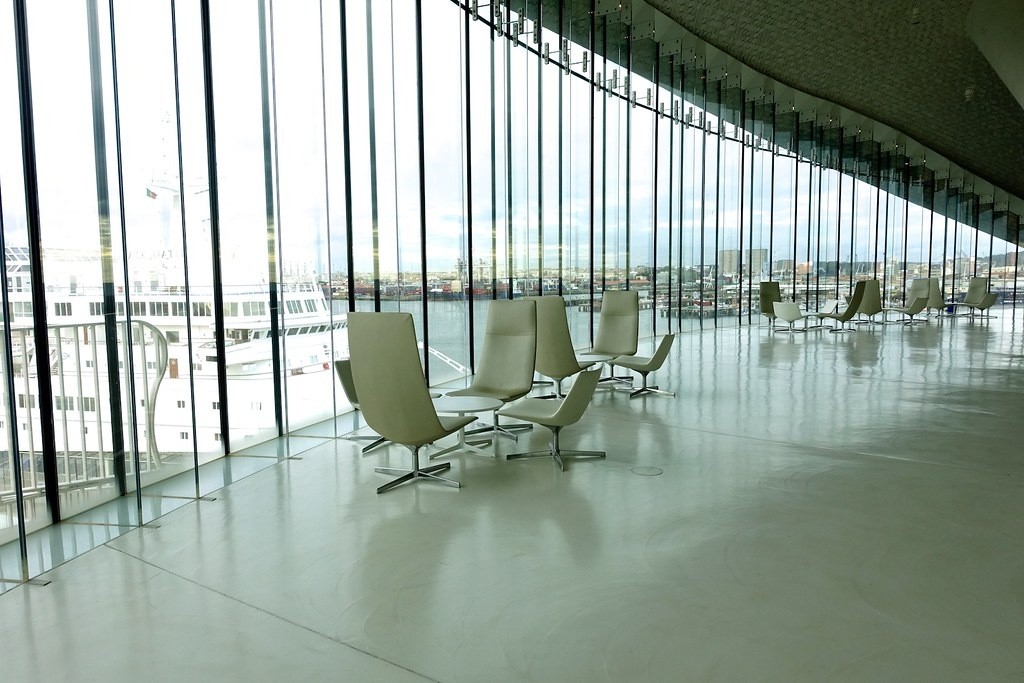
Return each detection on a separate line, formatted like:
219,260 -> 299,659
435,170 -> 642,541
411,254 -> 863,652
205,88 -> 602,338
806,312 -> 834,331
568,354 -> 618,394
427,396 -> 506,462
881,306 -> 899,324
942,302 -> 968,319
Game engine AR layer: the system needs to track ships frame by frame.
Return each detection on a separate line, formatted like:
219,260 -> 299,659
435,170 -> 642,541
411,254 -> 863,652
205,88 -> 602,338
0,162 -> 389,455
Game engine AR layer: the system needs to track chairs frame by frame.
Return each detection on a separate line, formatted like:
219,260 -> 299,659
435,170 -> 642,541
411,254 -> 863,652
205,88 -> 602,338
346,312 -> 480,495
962,293 -> 999,319
608,333 -> 678,399
579,290 -> 642,389
518,294 -> 596,403
807,299 -> 839,331
962,277 -> 993,316
336,360 -> 444,453
925,277 -> 954,320
771,301 -> 812,335
825,280 -> 867,333
855,279 -> 886,326
894,296 -> 929,328
758,281 -> 788,333
444,297 -> 540,444
896,278 -> 930,325
493,363 -> 610,472
